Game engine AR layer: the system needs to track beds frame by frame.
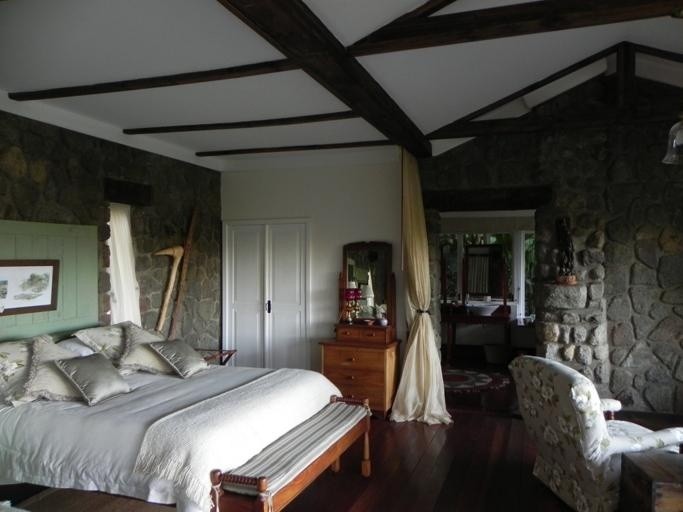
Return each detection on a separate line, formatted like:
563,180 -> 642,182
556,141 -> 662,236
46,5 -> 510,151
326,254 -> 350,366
1,327 -> 343,512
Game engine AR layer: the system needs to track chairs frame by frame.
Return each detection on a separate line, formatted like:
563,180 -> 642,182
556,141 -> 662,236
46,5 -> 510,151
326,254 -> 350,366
508,354 -> 683,512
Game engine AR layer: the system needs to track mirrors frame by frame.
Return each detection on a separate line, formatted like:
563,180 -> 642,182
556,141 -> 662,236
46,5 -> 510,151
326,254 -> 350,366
342,240 -> 393,324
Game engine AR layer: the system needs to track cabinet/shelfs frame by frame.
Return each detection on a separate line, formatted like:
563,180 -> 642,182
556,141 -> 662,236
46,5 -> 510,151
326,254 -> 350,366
335,322 -> 397,344
318,338 -> 402,419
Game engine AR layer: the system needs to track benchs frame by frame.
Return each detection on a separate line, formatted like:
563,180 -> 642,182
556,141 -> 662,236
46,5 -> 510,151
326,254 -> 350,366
209,395 -> 372,512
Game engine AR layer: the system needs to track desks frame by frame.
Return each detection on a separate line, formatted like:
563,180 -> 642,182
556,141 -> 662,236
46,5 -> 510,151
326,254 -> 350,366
618,451 -> 683,512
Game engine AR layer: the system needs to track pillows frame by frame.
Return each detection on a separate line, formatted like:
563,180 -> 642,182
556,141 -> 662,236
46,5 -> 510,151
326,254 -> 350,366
55,337 -> 94,357
149,338 -> 211,378
22,336 -> 88,402
0,335 -> 55,407
71,324 -> 125,361
53,353 -> 129,405
119,323 -> 174,374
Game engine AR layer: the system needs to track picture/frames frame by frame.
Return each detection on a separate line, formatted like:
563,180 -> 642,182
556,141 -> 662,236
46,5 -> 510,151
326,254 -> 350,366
0,259 -> 60,317
